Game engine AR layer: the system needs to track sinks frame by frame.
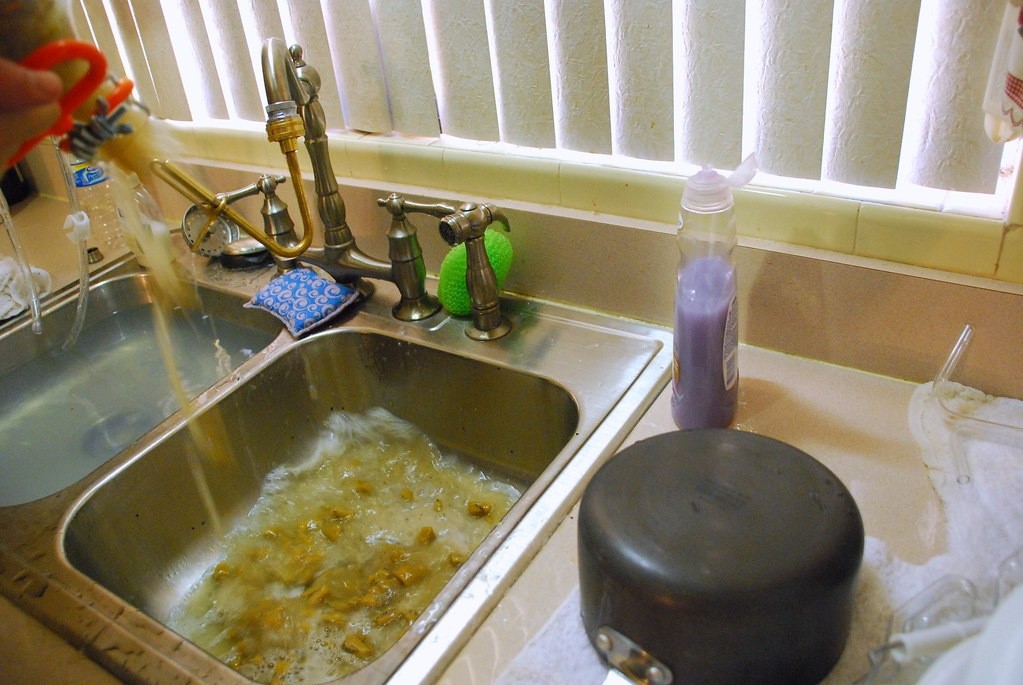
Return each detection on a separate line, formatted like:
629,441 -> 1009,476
56,328 -> 581,685
0,271 -> 287,510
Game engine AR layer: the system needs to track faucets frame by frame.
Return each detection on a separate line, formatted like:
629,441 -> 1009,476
437,197 -> 517,341
204,34 -> 452,327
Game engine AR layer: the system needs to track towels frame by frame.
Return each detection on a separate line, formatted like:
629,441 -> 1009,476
491,380 -> 1023,685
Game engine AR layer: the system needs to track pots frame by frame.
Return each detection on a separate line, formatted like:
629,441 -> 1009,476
577,429 -> 864,685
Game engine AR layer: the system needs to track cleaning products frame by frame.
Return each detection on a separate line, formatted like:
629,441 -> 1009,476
667,148 -> 763,432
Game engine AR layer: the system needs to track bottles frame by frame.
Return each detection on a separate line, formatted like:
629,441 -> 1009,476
66,152 -> 122,248
117,173 -> 173,266
670,152 -> 758,431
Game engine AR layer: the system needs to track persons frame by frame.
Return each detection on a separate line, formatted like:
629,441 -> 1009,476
0,0 -> 175,214
